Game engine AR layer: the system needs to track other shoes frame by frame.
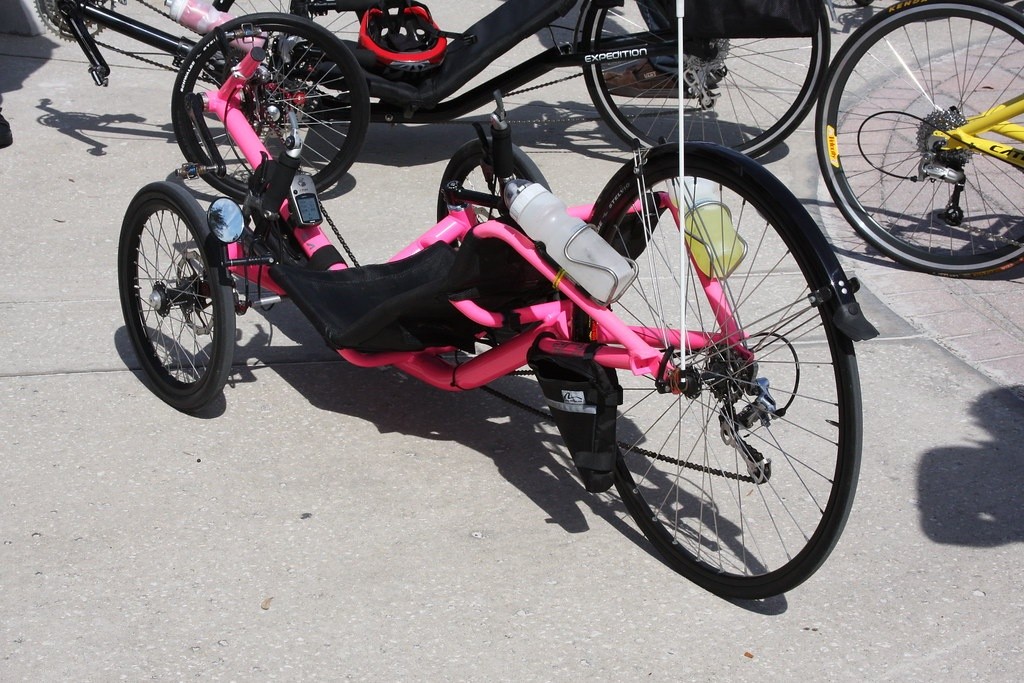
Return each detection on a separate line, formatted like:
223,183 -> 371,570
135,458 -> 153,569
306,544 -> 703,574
0,114 -> 13,147
602,59 -> 694,98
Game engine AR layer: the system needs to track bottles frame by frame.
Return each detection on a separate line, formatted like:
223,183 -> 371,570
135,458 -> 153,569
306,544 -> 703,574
667,177 -> 742,278
501,176 -> 634,301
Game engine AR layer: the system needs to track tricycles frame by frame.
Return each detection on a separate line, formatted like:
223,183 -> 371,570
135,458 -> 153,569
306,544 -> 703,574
1,0 -> 1024,598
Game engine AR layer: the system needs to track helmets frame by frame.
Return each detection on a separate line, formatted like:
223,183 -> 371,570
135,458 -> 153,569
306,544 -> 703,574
359,7 -> 447,71
